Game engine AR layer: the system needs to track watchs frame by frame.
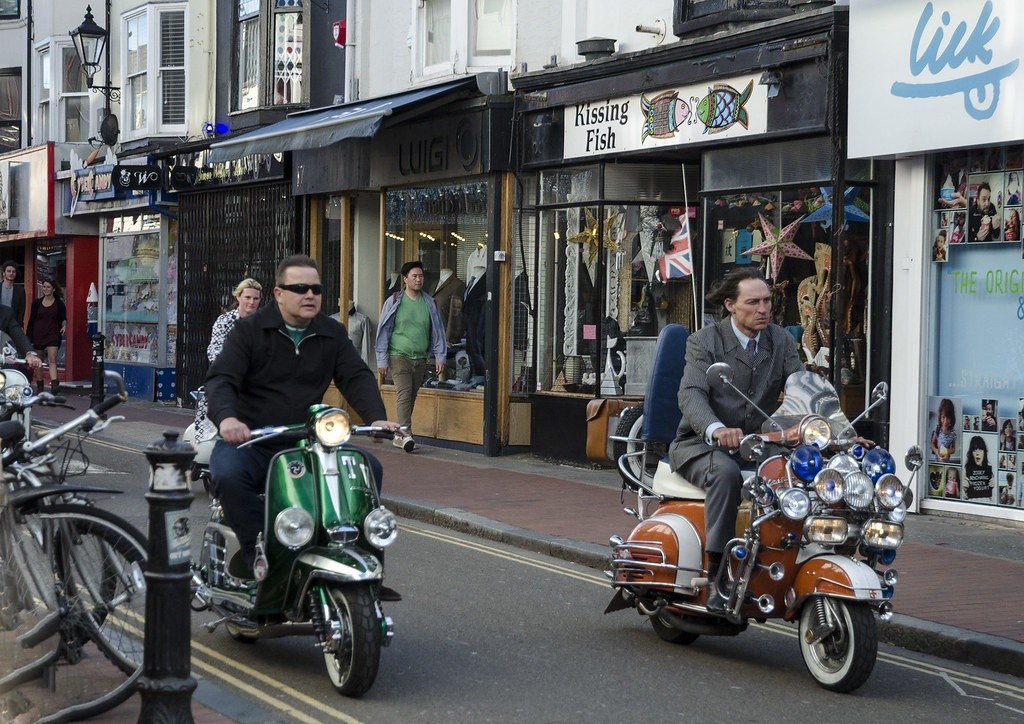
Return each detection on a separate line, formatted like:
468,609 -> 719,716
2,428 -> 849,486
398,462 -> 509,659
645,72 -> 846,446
26,351 -> 40,358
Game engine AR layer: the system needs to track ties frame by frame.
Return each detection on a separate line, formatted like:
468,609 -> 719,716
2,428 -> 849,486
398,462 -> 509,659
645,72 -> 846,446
477,245 -> 482,257
463,276 -> 475,302
747,339 -> 757,364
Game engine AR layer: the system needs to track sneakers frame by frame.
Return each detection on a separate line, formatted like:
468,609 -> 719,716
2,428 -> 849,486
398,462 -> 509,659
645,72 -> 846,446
392,434 -> 416,452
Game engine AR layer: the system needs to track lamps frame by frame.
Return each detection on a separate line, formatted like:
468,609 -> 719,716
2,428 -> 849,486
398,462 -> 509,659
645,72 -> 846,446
533,114 -> 554,127
759,69 -> 784,86
69,5 -> 121,105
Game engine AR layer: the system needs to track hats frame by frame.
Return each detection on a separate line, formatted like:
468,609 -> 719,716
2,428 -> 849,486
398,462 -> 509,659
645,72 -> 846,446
985,400 -> 992,407
401,261 -> 423,275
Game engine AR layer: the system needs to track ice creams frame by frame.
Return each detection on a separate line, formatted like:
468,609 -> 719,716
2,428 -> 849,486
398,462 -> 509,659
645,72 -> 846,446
981,214 -> 991,228
954,225 -> 960,234
941,174 -> 954,200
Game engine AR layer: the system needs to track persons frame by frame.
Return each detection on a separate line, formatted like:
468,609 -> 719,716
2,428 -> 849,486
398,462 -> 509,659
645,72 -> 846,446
327,297 -> 371,370
375,261 -> 447,453
384,240 -> 487,368
0,304 -> 42,369
670,268 -> 876,615
26,279 -> 67,396
514,267 -> 529,354
931,398 -> 1024,507
0,263 -> 26,331
204,255 -> 405,602
931,146 -> 1020,262
195,278 -> 263,444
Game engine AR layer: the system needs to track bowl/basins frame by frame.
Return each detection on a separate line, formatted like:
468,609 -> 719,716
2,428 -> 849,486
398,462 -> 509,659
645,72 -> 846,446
942,189 -> 954,200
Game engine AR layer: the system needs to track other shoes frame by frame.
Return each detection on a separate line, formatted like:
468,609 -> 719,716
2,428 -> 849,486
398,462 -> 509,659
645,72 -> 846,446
379,584 -> 402,600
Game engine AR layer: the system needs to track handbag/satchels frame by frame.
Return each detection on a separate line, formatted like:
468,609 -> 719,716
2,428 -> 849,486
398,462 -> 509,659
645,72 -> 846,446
836,333 -> 867,385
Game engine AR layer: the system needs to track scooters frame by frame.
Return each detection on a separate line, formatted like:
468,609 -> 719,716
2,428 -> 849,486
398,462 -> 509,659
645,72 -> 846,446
603,323 -> 924,693
0,331 -> 76,553
183,386 -> 220,493
191,402 -> 396,697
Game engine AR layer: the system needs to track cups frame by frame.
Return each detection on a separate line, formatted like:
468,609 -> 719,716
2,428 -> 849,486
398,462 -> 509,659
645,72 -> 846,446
947,480 -> 955,494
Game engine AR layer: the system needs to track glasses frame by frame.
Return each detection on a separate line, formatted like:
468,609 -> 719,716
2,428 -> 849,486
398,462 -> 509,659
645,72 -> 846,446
278,283 -> 324,295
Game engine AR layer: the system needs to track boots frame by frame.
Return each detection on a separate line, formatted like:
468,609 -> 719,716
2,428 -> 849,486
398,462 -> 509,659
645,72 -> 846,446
706,562 -> 728,611
51,379 -> 62,396
36,381 -> 49,401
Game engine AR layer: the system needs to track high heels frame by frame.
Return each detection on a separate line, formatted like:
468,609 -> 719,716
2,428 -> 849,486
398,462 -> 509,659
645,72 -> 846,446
512,376 -> 523,393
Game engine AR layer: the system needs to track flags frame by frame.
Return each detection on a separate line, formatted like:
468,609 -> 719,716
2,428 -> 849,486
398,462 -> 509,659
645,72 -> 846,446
658,213 -> 693,280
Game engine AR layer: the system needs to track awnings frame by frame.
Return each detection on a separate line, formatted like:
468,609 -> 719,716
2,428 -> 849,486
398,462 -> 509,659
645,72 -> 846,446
208,77 -> 475,164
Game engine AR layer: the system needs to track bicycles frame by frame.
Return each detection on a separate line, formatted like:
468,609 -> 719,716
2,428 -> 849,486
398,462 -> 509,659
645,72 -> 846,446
0,369 -> 148,677
